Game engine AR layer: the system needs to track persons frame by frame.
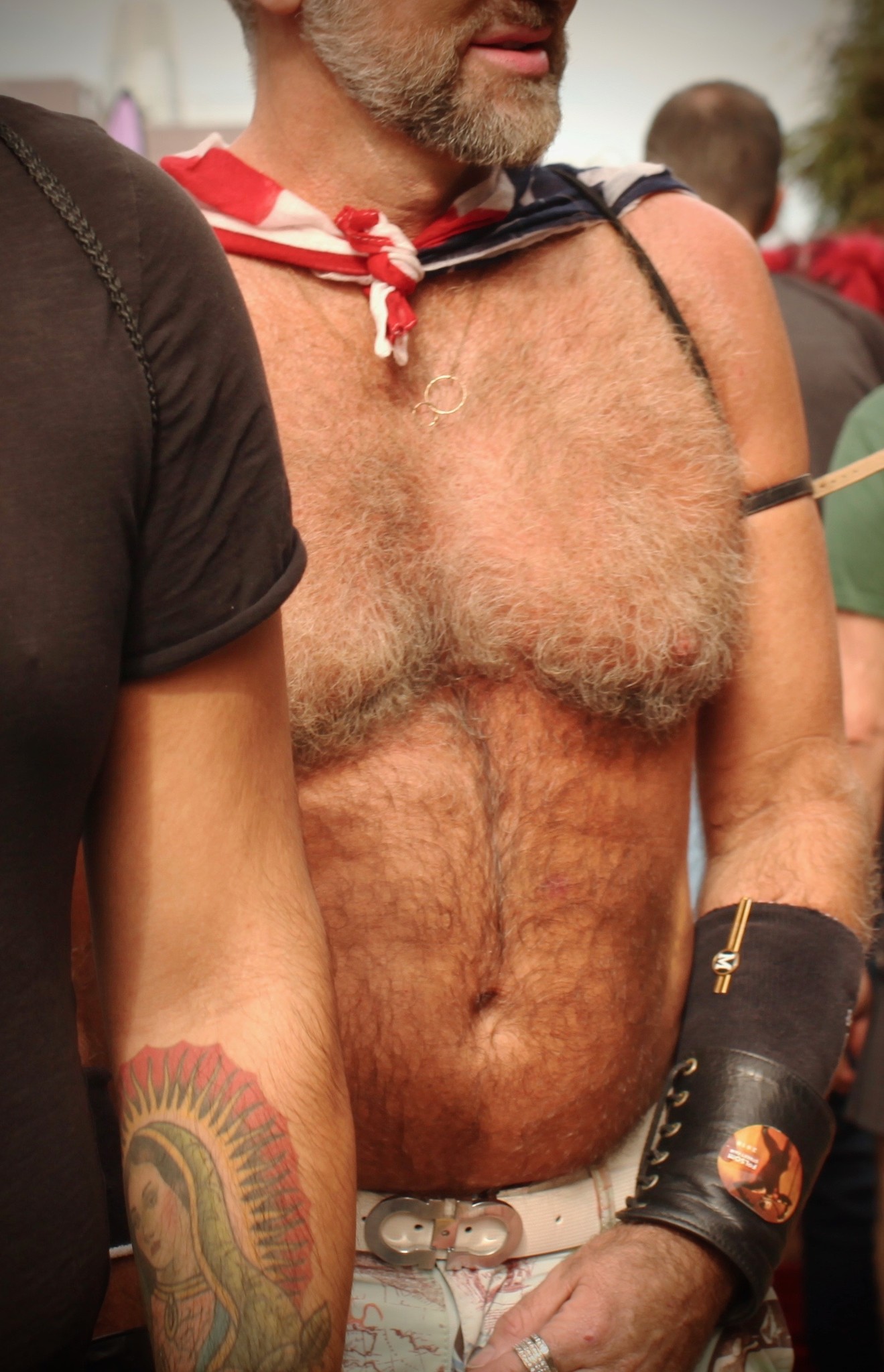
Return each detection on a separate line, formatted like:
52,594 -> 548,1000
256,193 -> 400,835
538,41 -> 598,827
0,95 -> 357,1372
802,388 -> 884,1372
146,0 -> 858,1372
648,83 -> 884,523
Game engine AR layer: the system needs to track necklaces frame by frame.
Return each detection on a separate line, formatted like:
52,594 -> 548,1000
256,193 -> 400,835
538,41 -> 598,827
302,259 -> 497,433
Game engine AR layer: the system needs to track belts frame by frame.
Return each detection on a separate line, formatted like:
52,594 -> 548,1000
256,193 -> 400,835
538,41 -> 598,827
354,1112 -> 647,1272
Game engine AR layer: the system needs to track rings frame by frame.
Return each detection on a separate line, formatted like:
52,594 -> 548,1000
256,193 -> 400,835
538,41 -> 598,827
511,1334 -> 559,1372
